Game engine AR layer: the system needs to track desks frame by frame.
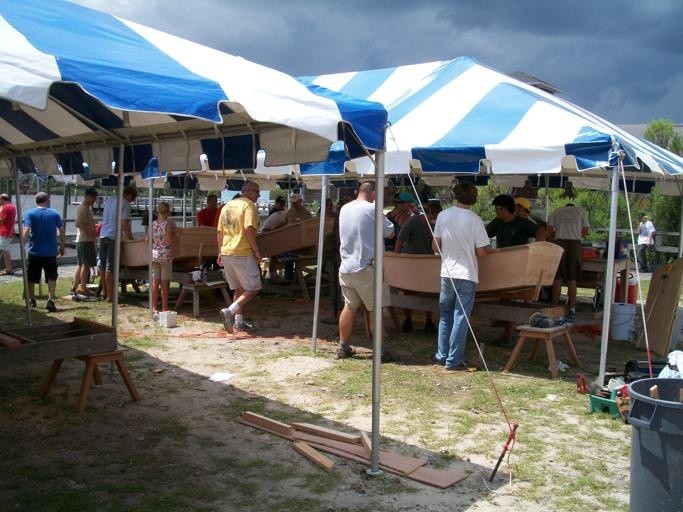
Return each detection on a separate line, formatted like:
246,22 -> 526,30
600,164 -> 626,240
561,256 -> 631,320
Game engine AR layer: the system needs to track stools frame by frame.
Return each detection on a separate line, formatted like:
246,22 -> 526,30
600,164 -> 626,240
40,345 -> 140,416
503,323 -> 580,381
287,259 -> 330,301
95,278 -> 141,298
173,281 -> 233,318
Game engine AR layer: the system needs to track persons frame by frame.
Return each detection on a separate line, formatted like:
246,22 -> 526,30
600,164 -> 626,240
316,178 -> 443,364
635,211 -> 656,273
259,188 -> 316,281
430,181 -> 594,373
145,201 -> 183,313
1,194 -> 18,276
21,190 -> 66,312
70,186 -> 138,302
198,178 -> 264,331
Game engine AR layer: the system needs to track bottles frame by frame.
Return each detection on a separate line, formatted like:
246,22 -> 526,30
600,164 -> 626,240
153,310 -> 159,322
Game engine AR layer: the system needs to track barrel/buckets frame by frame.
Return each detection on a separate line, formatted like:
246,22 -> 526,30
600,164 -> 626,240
617,278 -> 638,303
626,378 -> 683,512
611,302 -> 638,342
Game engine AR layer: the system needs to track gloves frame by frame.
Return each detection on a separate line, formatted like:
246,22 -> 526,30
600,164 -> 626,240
615,395 -> 633,424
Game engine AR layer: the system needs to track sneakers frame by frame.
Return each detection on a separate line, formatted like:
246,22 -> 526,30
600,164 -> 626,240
401,320 -> 412,331
233,321 -> 256,332
567,310 -> 576,319
46,300 -> 57,312
219,308 -> 234,333
28,299 -> 36,307
336,345 -> 355,358
380,351 -> 400,363
431,353 -> 469,370
71,283 -> 94,296
425,322 -> 437,333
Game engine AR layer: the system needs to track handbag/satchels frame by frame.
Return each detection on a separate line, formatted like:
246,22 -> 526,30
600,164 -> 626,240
401,238 -> 434,254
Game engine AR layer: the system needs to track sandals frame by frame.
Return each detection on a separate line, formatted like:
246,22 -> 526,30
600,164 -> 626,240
0,268 -> 14,275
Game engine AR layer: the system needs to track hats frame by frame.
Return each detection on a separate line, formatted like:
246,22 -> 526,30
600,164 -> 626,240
514,197 -> 531,211
290,194 -> 302,202
392,192 -> 416,206
276,197 -> 285,203
